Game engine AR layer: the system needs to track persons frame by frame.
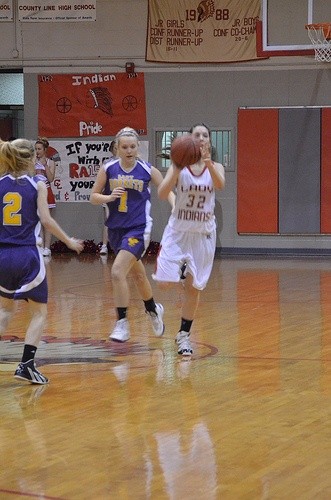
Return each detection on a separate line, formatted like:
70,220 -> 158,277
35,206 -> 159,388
152,122 -> 225,356
31,138 -> 56,256
101,141 -> 114,255
0,140 -> 85,384
90,127 -> 177,342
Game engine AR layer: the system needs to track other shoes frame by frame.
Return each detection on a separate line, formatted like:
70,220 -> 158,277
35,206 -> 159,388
99,245 -> 108,254
40,247 -> 51,257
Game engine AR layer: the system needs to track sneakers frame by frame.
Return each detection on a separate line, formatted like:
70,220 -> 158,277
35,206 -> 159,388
13,359 -> 49,385
109,316 -> 132,343
174,330 -> 193,356
145,303 -> 165,338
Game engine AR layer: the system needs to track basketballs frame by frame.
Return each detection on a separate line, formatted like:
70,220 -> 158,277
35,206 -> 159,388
171,134 -> 201,166
